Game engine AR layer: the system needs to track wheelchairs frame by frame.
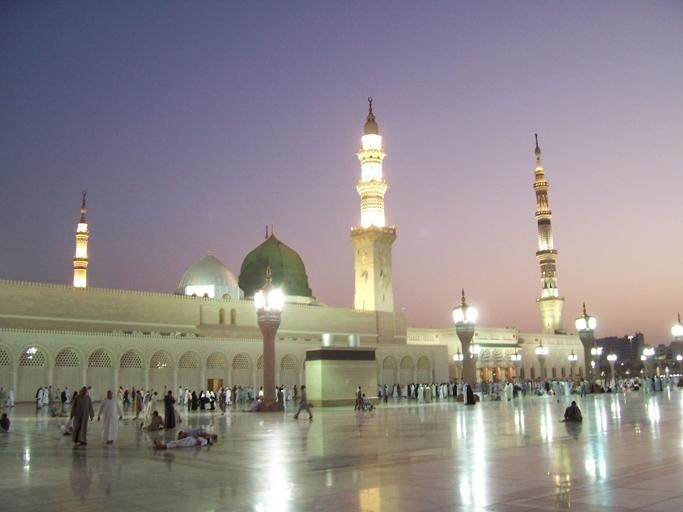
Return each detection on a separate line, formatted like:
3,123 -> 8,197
359,396 -> 376,411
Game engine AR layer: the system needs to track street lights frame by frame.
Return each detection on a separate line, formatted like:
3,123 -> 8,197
573,301 -> 601,392
452,335 -> 683,394
451,286 -> 479,401
671,312 -> 683,388
252,267 -> 287,412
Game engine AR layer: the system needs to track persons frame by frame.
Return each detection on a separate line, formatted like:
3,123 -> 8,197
118,384 -> 182,431
353,385 -> 376,410
0,388 -> 12,431
35,385 -> 71,418
97,388 -> 123,444
153,428 -> 218,450
462,382 -> 476,405
185,383 -> 297,414
590,376 -> 674,394
58,382 -> 95,446
294,385 -> 313,419
476,378 -> 586,403
377,383 -> 463,403
564,400 -> 583,424
